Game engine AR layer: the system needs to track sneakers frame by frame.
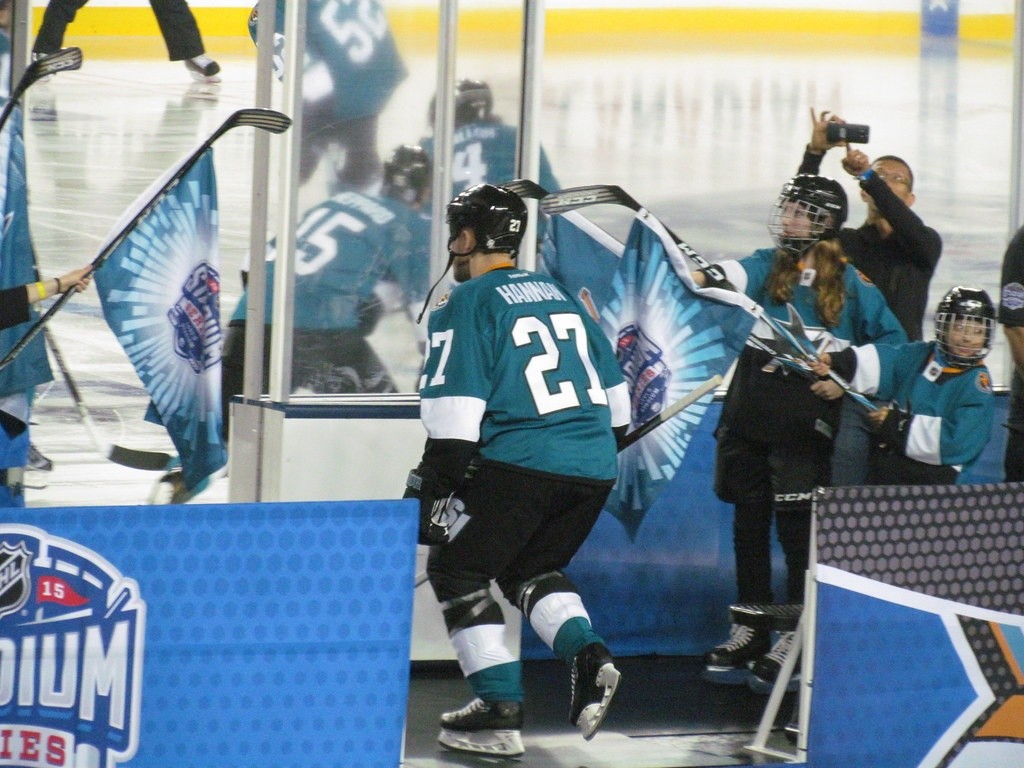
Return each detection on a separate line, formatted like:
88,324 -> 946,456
751,630 -> 802,693
24,445 -> 53,490
437,698 -> 525,757
30,52 -> 56,82
569,642 -> 622,742
185,54 -> 221,83
705,624 -> 770,684
150,463 -> 194,504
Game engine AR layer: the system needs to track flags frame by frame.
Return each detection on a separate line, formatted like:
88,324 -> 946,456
553,218 -> 620,327
88,143 -> 230,490
600,219 -> 761,541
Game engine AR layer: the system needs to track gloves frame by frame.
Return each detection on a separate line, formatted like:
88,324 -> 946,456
402,462 -> 458,546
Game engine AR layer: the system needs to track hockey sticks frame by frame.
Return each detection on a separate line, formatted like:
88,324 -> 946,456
538,183 -> 878,413
0,45 -> 83,133
414,373 -> 724,589
0,107 -> 294,371
38,310 -> 174,471
498,178 -> 819,384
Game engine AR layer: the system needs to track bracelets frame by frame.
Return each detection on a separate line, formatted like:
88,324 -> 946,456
54,277 -> 61,293
35,282 -> 47,299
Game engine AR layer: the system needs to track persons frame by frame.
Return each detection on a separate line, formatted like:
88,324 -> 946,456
0,266 -> 93,329
29,0 -> 221,84
396,188 -> 625,754
248,0 -> 413,197
686,173 -> 909,688
418,92 -> 563,216
800,282 -> 996,484
789,106 -> 945,483
996,224 -> 1023,485
150,142 -> 432,503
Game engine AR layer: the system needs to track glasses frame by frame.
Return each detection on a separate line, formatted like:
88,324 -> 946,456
876,171 -> 911,194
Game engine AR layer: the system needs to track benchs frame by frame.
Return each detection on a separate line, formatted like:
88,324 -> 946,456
729,485 -> 1024,740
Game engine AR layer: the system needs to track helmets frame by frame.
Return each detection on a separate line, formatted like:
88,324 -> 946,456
447,183 -> 527,259
383,146 -> 431,190
767,173 -> 847,254
934,285 -> 996,369
455,79 -> 492,119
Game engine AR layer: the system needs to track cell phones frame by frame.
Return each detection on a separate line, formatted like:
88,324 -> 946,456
825,121 -> 869,144
